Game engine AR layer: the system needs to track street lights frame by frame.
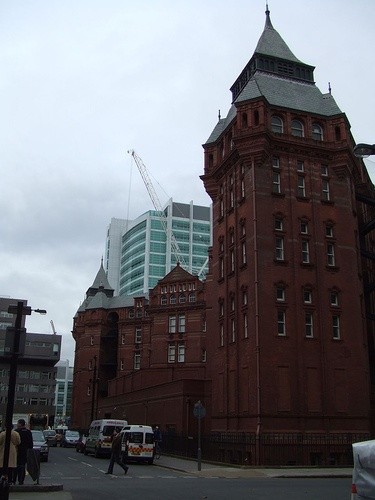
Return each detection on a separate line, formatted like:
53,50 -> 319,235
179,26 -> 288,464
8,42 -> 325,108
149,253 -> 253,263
0,302 -> 47,500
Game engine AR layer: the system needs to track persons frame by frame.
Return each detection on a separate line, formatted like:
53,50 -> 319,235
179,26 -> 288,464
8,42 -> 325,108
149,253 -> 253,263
104,428 -> 129,475
153,426 -> 160,441
12,419 -> 33,485
0,424 -> 21,483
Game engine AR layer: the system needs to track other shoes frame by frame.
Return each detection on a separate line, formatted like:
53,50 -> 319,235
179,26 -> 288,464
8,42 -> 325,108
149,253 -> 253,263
105,472 -> 113,475
125,467 -> 129,475
19,481 -> 23,485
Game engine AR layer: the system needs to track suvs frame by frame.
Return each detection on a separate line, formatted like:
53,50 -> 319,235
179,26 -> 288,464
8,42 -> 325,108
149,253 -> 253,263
31,430 -> 49,462
43,429 -> 81,447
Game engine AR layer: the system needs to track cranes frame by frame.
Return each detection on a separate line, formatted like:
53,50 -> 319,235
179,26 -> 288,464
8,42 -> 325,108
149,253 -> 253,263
125,149 -> 209,271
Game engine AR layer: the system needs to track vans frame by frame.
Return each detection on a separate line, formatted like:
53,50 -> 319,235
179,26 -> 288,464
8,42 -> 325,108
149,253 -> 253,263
83,419 -> 128,458
119,425 -> 155,465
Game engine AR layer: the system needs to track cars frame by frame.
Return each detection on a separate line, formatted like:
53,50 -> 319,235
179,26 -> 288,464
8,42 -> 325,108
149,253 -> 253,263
75,433 -> 88,453
